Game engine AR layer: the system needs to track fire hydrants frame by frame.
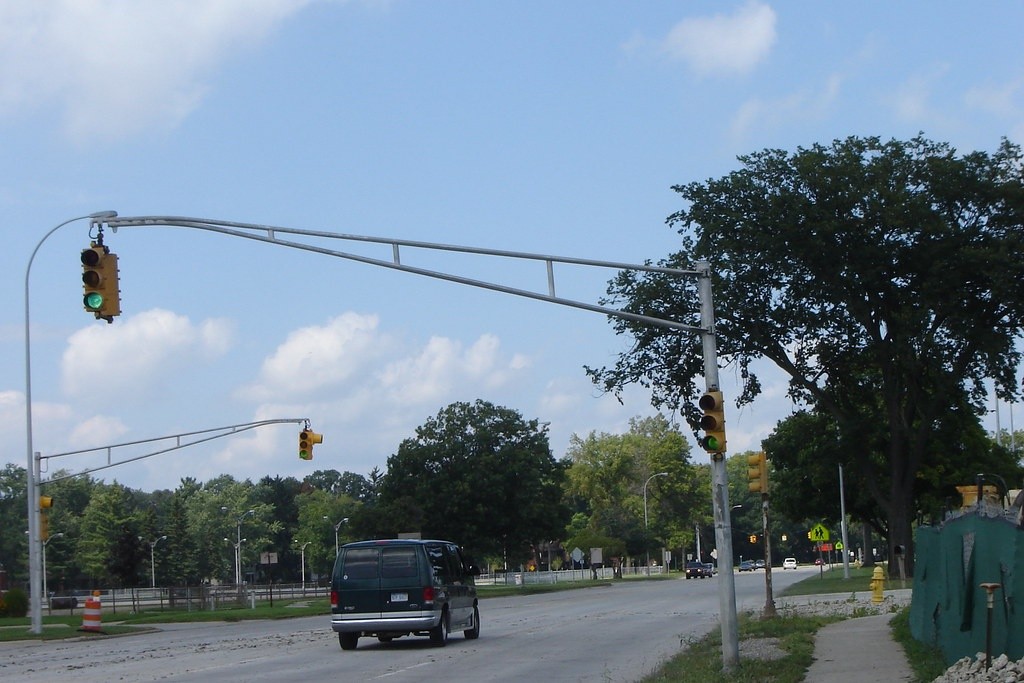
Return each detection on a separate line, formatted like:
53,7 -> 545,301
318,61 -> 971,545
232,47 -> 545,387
869,566 -> 886,606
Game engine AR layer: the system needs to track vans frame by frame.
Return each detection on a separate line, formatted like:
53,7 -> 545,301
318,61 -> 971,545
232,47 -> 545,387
329,540 -> 480,650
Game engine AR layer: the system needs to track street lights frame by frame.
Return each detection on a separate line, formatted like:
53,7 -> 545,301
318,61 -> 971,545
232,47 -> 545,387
978,473 -> 1012,508
324,517 -> 350,557
220,508 -> 255,590
138,536 -> 167,598
25,211 -> 119,633
294,541 -> 313,592
645,471 -> 669,576
26,531 -> 63,597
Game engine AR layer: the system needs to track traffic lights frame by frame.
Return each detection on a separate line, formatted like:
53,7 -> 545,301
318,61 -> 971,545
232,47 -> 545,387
748,453 -> 767,493
38,495 -> 55,510
300,429 -> 322,461
700,392 -> 728,453
82,245 -> 108,315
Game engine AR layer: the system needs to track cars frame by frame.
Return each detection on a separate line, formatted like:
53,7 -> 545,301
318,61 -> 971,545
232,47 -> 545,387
815,558 -> 825,565
782,558 -> 797,570
51,590 -> 77,608
739,559 -> 766,572
685,562 -> 715,579
